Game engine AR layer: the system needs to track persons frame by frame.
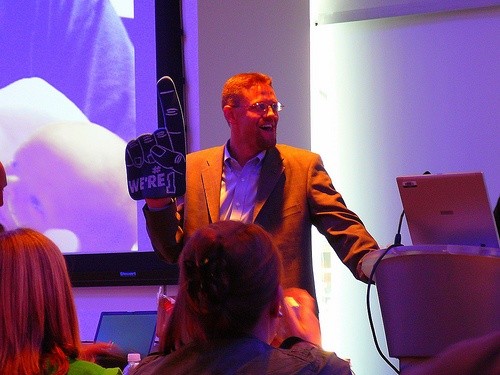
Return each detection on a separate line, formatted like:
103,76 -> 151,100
0,160 -> 125,375
128,220 -> 353,375
142,72 -> 379,333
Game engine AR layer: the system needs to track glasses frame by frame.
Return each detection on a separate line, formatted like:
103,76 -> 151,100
235,101 -> 285,112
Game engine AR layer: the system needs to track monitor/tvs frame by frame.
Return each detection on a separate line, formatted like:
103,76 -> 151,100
92,311 -> 158,359
395,171 -> 500,248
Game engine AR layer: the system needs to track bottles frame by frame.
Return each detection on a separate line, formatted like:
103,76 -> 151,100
122,353 -> 142,375
